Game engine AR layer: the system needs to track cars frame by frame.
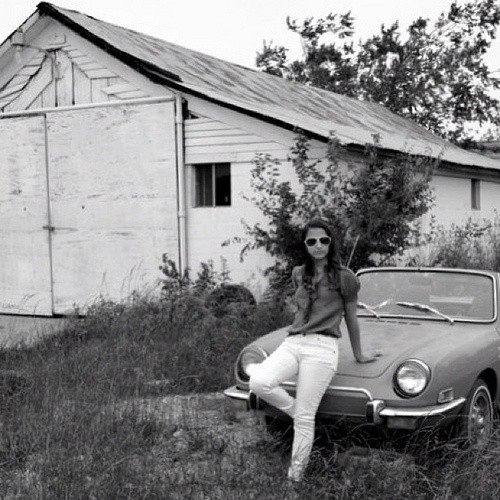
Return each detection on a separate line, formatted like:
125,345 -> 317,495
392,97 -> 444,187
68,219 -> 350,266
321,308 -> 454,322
222,264 -> 498,454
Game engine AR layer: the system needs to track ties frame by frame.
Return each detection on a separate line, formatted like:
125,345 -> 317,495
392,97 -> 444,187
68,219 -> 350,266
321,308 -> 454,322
302,283 -> 318,325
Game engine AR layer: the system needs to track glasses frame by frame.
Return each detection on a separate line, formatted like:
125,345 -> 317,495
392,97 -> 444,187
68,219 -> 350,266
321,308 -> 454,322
304,236 -> 332,248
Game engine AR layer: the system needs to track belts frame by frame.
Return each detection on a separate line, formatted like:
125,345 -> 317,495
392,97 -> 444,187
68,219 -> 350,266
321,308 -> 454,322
289,329 -> 339,338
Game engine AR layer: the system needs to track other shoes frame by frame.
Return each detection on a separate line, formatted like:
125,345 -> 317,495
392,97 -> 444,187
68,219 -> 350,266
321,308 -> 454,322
319,424 -> 333,456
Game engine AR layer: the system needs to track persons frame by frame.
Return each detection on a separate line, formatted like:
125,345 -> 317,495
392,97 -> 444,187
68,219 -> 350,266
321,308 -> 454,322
248,217 -> 386,487
